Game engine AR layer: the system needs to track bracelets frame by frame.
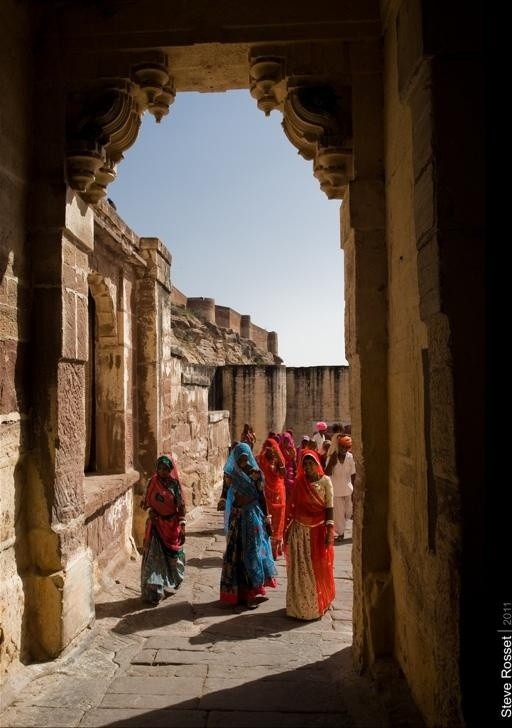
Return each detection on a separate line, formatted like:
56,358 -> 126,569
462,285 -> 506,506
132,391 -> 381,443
142,504 -> 145,510
179,517 -> 186,520
146,507 -> 152,514
179,520 -> 186,526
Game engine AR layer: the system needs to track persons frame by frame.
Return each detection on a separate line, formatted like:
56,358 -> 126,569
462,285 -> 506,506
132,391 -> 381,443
230,419 -> 356,560
282,449 -> 336,621
139,450 -> 188,607
217,442 -> 279,614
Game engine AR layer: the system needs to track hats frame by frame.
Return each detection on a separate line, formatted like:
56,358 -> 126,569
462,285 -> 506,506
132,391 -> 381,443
339,436 -> 352,448
316,422 -> 328,431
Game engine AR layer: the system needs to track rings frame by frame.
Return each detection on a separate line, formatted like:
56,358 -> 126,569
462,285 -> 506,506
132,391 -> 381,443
156,517 -> 158,520
